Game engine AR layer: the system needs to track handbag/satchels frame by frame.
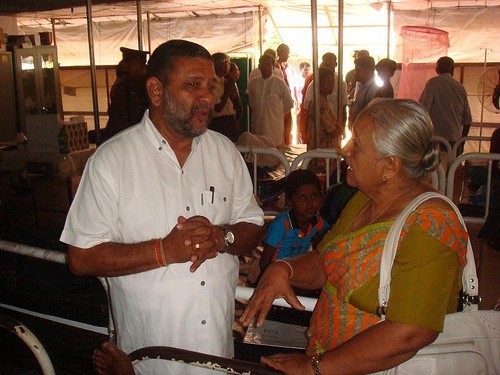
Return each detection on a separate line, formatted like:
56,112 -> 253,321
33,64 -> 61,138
369,191 -> 499,374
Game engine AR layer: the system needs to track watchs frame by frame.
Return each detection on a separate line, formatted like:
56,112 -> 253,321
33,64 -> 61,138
220,224 -> 236,253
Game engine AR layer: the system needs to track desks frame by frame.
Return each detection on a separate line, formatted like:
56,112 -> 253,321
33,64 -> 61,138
0,141 -> 103,232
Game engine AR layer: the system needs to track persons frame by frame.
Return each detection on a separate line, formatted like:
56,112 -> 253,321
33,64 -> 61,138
247,42 -> 397,165
105,45 -> 150,136
419,56 -> 473,157
240,97 -> 469,375
91,341 -> 135,374
245,56 -> 296,147
210,52 -> 244,140
261,169 -> 333,272
60,39 -> 266,374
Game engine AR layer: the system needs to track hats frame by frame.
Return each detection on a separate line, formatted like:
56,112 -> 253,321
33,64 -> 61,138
120,47 -> 150,64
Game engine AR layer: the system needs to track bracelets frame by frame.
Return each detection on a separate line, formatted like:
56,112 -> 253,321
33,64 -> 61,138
272,258 -> 294,280
309,352 -> 323,373
154,237 -> 168,270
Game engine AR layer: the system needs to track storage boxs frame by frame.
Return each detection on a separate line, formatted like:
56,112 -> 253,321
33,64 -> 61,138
59,121 -> 89,151
